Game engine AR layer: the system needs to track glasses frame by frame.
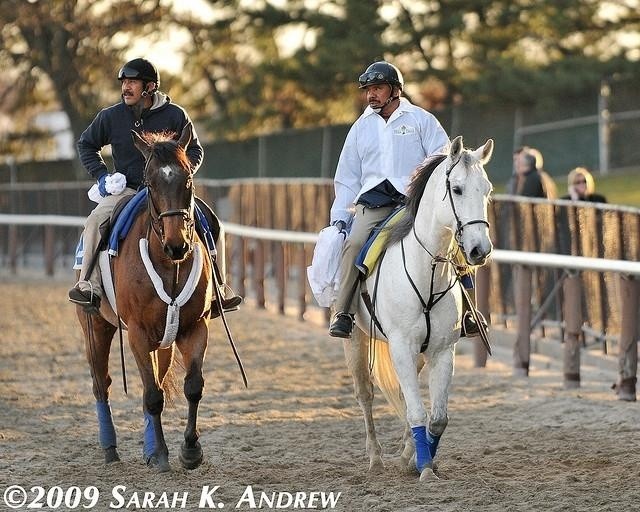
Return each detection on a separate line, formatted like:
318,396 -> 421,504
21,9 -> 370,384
118,67 -> 141,79
359,72 -> 387,86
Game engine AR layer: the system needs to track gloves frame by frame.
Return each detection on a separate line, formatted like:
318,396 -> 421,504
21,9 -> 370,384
98,174 -> 112,198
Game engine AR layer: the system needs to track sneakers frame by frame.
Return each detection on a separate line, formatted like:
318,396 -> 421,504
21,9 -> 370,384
69,287 -> 101,315
460,316 -> 487,338
204,295 -> 242,320
329,311 -> 355,339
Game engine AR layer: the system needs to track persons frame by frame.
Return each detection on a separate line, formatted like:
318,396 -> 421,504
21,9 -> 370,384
327,61 -> 489,341
560,165 -> 609,202
515,148 -> 560,200
505,145 -> 531,194
67,57 -> 244,319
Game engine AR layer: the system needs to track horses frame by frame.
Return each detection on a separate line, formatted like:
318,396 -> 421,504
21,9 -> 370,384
342,136 -> 495,484
75,121 -> 213,474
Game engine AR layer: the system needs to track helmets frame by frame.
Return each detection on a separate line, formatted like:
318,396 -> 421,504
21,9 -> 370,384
358,61 -> 403,91
118,58 -> 161,84
